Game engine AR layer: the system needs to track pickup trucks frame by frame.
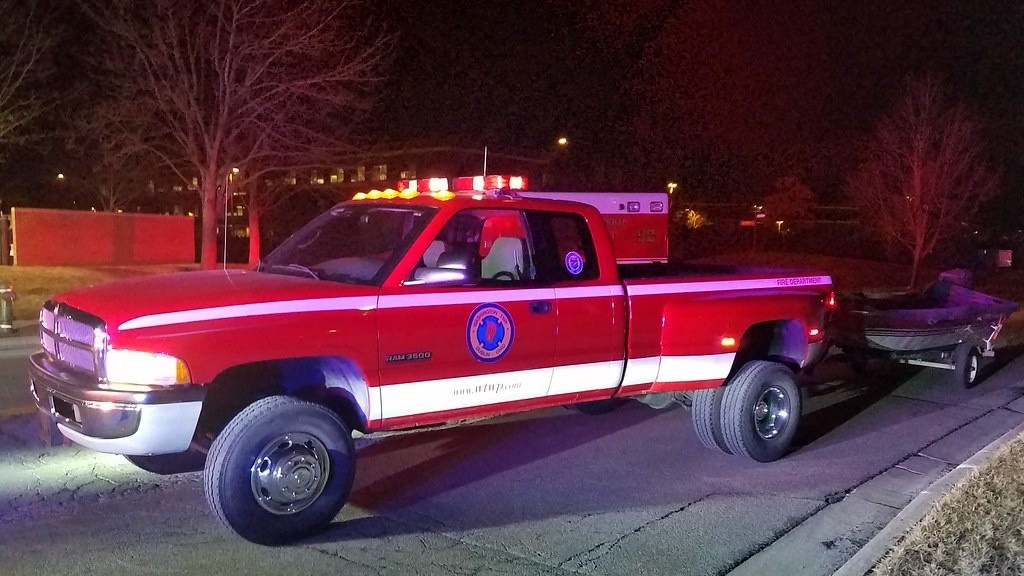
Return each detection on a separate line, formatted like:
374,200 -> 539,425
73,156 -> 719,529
26,172 -> 839,546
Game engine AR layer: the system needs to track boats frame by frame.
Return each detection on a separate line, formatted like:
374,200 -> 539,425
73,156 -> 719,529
823,266 -> 1024,356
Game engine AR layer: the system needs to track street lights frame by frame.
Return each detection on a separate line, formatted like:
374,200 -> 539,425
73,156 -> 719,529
222,165 -> 241,268
549,136 -> 569,191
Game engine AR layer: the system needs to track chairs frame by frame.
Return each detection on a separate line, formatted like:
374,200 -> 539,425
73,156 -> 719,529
415,240 -> 452,279
478,213 -> 536,257
481,236 -> 529,282
